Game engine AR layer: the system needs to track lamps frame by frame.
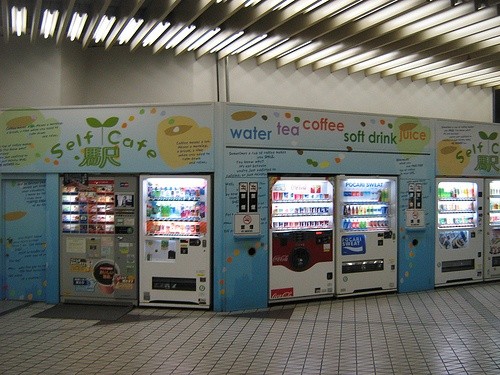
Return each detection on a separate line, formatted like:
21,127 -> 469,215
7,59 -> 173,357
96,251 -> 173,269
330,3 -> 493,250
10,5 -> 497,90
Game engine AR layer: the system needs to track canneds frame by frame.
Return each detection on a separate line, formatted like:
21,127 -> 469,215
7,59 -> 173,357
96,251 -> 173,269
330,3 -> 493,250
298,207 -> 330,213
273,191 -> 329,200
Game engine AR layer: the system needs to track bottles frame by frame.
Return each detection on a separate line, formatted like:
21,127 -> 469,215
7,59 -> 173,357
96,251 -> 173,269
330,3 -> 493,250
438,185 -> 476,210
148,184 -> 205,236
344,186 -> 388,228
